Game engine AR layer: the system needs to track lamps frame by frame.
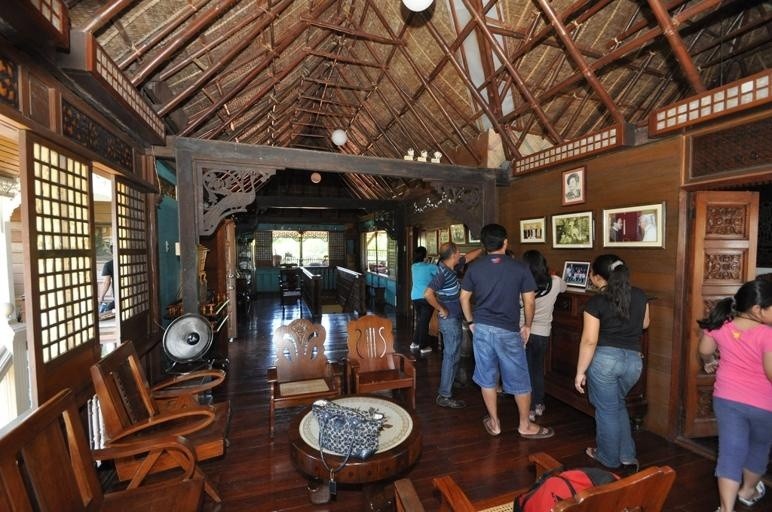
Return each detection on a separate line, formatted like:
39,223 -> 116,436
331,113 -> 348,145
311,173 -> 321,183
403,0 -> 433,12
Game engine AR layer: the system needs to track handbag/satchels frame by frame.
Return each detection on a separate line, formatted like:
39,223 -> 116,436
308,399 -> 385,475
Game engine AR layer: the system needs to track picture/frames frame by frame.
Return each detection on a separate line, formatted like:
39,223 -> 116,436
600,201 -> 666,249
550,209 -> 596,250
561,257 -> 591,289
450,222 -> 467,247
518,216 -> 547,245
420,228 -> 438,257
560,164 -> 587,207
438,226 -> 452,254
467,227 -> 482,246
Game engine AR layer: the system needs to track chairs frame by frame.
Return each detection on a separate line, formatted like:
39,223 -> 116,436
393,451 -> 676,512
340,314 -> 417,411
280,263 -> 298,270
91,339 -> 233,503
266,318 -> 341,440
279,269 -> 302,320
0,388 -> 223,512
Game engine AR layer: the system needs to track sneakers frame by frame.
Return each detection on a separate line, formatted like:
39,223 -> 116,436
529,411 -> 536,421
420,346 -> 433,353
433,391 -> 457,403
409,343 -> 419,348
437,396 -> 466,408
535,404 -> 546,417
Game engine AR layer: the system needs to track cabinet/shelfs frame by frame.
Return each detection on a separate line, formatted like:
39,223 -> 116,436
236,231 -> 257,330
543,290 -> 657,433
164,299 -> 231,392
156,359 -> 216,404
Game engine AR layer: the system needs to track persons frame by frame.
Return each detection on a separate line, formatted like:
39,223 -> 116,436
424,240 -> 486,409
410,245 -> 437,353
459,223 -> 554,440
99,246 -> 114,304
609,215 -> 623,241
521,250 -> 566,422
566,266 -> 586,284
696,279 -> 771,512
575,253 -> 651,473
565,173 -> 582,199
639,213 -> 657,241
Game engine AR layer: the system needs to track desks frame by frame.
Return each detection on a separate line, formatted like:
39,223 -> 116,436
278,274 -> 301,306
288,392 -> 424,512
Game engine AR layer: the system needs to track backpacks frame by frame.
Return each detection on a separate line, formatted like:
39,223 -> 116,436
513,465 -> 631,512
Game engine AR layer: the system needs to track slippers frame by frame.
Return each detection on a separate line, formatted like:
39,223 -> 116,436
482,416 -> 502,436
520,424 -> 555,438
586,446 -> 596,457
739,481 -> 765,505
